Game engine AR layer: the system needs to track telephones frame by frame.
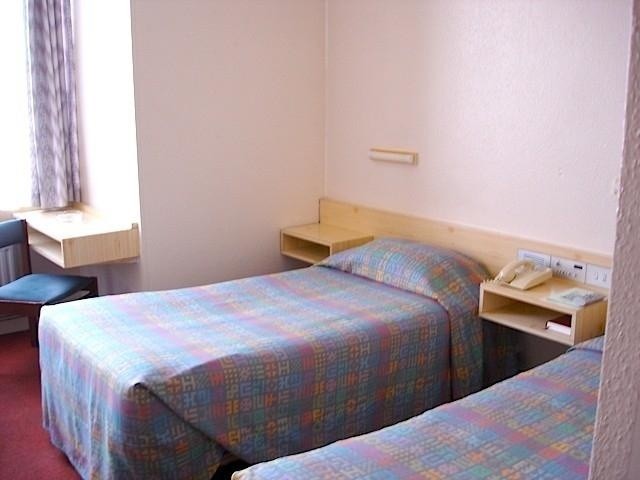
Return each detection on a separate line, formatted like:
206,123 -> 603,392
499,258 -> 552,290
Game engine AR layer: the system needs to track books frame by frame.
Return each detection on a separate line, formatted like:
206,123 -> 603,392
545,316 -> 571,336
551,286 -> 606,306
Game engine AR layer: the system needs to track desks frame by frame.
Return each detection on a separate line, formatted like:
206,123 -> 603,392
478,273 -> 608,347
278,223 -> 374,264
12,205 -> 141,271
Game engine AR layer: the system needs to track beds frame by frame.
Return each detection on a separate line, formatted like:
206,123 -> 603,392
36,243 -> 492,479
231,332 -> 605,479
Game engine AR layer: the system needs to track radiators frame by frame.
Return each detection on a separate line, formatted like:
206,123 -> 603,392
0,243 -> 30,288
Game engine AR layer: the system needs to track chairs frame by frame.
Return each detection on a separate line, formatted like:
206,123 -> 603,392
0,218 -> 101,347
